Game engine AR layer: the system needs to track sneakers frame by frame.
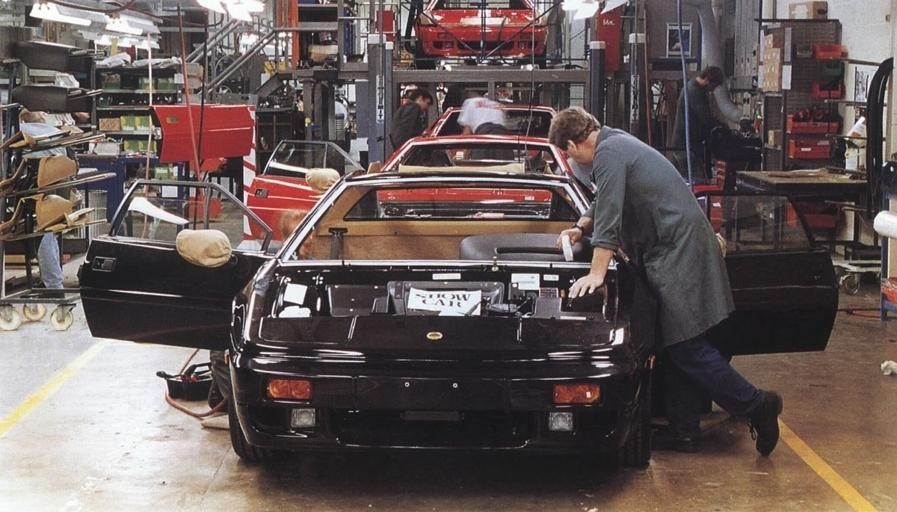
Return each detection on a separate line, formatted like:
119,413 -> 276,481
747,390 -> 784,456
651,425 -> 704,454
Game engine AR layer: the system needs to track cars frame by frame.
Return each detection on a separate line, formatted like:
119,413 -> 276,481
411,0 -> 548,71
75,102 -> 840,469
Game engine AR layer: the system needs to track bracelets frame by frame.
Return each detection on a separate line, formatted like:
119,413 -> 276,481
573,224 -> 584,234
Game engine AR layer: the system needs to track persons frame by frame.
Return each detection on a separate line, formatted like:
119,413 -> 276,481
456,94 -> 514,166
548,106 -> 788,455
385,88 -> 434,159
19,102 -> 88,175
268,208 -> 319,259
670,66 -> 730,180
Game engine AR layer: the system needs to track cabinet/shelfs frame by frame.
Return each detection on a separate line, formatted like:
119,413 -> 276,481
2,38 -> 115,308
761,23 -> 839,250
